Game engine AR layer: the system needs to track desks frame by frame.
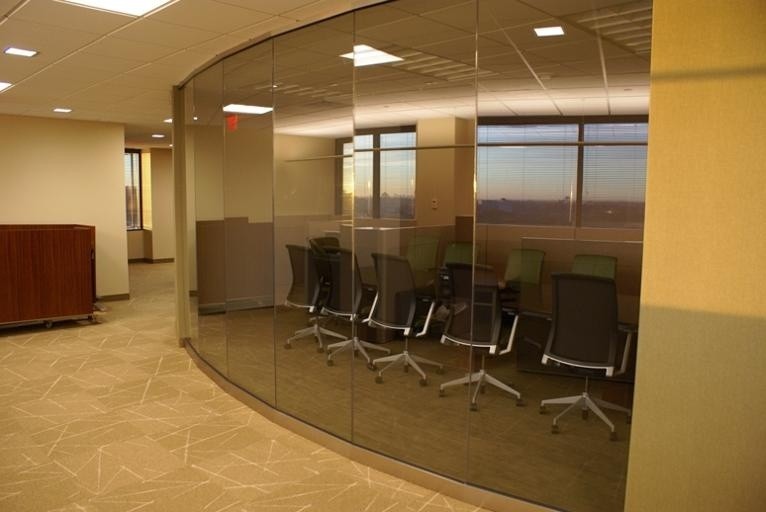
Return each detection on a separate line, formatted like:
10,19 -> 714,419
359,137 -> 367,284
340,264 -> 639,384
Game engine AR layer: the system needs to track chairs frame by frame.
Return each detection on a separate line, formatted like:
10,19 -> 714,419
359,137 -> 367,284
284,244 -> 351,353
411,240 -> 480,338
399,236 -> 440,335
496,249 -> 545,362
539,271 -> 633,441
553,254 -> 618,279
361,252 -> 448,387
320,246 -> 392,368
439,262 -> 524,411
304,237 -> 351,329
